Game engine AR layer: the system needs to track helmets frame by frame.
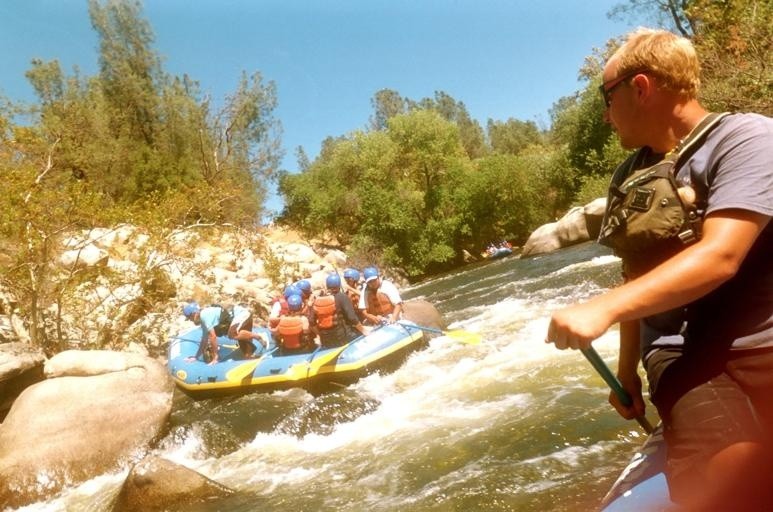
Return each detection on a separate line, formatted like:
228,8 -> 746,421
296,280 -> 311,292
182,302 -> 200,321
284,284 -> 303,298
286,295 -> 303,311
325,273 -> 341,288
344,269 -> 360,282
363,267 -> 377,283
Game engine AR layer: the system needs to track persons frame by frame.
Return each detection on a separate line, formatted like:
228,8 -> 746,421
180,267 -> 405,366
546,27 -> 770,511
484,238 -> 513,255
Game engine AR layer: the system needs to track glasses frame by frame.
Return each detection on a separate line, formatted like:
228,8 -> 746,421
596,67 -> 651,109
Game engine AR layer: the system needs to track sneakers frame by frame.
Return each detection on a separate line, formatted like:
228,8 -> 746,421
257,331 -> 269,353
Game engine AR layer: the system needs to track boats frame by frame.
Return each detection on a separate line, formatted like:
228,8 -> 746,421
168,320 -> 424,401
488,248 -> 512,260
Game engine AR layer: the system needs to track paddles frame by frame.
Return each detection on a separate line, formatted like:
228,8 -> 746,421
283,344 -> 322,380
168,335 -> 244,355
383,319 -> 483,346
225,346 -> 280,383
307,320 -> 388,369
